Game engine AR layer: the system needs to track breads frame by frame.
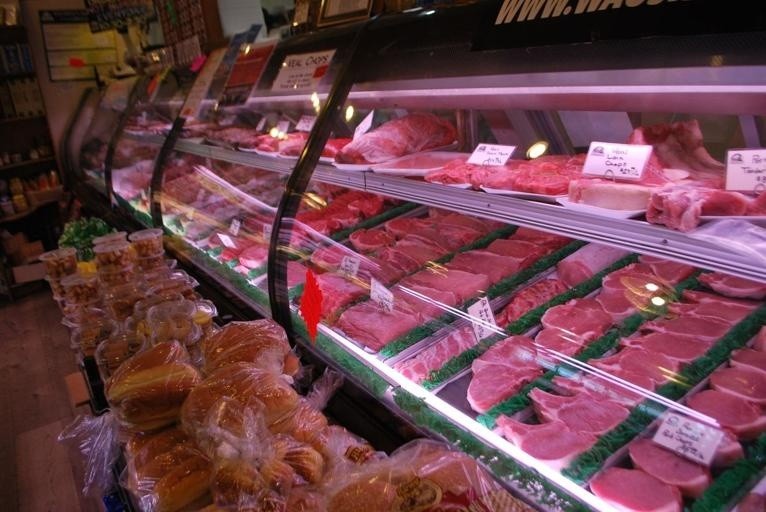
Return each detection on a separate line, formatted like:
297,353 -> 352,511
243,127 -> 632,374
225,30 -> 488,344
102,319 -> 529,512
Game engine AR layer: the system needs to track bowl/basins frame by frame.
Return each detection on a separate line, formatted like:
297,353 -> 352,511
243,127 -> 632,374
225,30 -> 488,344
38,229 -> 218,375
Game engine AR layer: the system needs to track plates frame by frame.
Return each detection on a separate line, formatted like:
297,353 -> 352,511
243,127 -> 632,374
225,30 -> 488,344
110,125 -> 766,279
